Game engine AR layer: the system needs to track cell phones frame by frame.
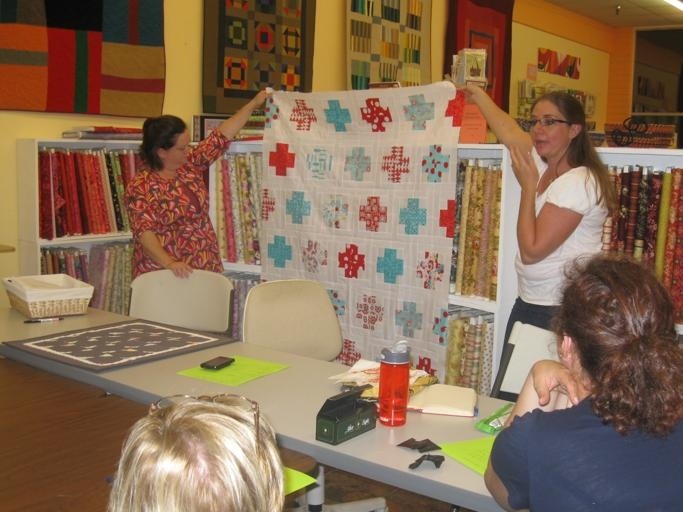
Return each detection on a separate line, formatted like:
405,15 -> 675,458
200,356 -> 235,370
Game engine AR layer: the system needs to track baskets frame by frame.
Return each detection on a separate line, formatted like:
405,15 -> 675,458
4,274 -> 95,318
604,122 -> 675,149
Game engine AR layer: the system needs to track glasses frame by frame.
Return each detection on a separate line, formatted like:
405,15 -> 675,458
149,392 -> 259,458
530,118 -> 569,126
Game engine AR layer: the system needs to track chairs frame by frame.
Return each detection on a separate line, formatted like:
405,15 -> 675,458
128,269 -> 235,337
243,279 -> 343,361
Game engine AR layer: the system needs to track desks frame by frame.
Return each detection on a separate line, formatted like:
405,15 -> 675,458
0,308 -> 514,512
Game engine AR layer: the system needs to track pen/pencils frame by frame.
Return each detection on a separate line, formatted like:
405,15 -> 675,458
24,318 -> 64,323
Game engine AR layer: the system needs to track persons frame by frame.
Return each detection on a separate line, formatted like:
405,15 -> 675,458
443,72 -> 620,406
483,252 -> 682,511
121,87 -> 272,279
107,392 -> 286,512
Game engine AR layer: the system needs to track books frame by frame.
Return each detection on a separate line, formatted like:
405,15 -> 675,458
375,383 -> 477,418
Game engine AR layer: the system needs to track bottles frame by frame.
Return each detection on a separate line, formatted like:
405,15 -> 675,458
376,345 -> 410,427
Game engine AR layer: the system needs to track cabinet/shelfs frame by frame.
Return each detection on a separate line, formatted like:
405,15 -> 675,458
15,138 -> 211,316
209,141 -> 683,402
